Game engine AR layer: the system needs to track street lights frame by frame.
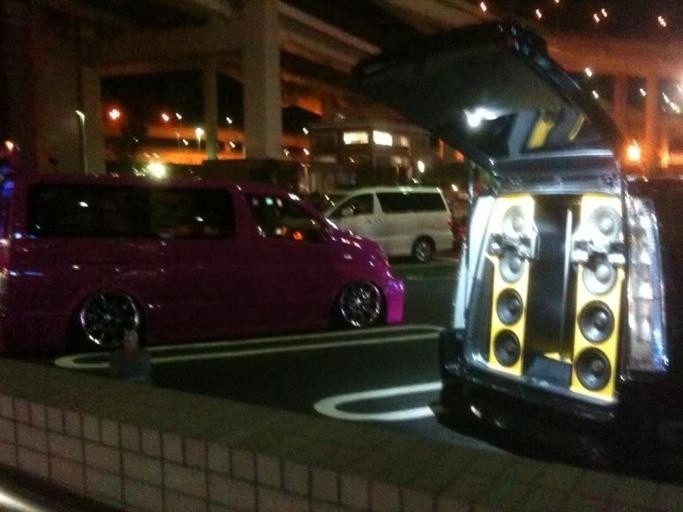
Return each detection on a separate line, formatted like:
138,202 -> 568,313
284,128 -> 309,171
109,107 -> 236,167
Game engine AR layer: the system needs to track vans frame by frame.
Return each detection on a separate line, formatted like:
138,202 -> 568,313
347,22 -> 681,435
1,173 -> 405,352
307,183 -> 455,264
